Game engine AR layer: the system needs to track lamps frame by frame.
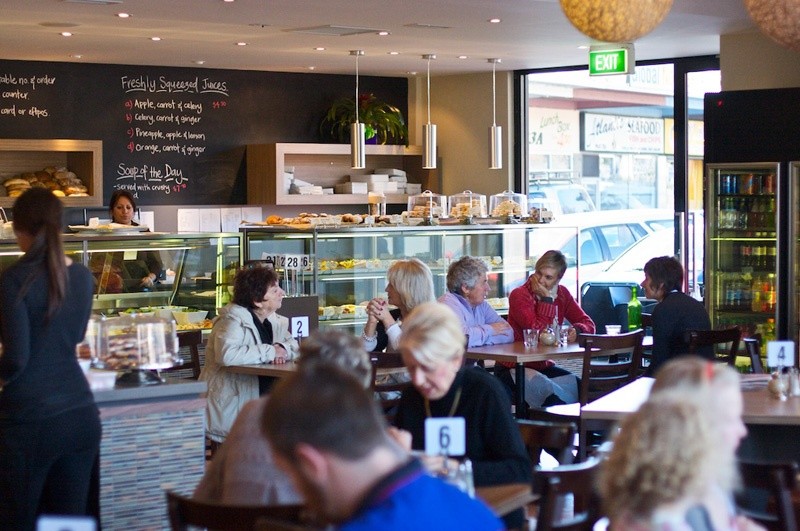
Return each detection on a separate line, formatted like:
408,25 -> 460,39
346,50 -> 367,171
420,54 -> 441,171
487,57 -> 504,170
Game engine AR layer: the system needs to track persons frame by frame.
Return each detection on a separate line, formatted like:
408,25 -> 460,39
506,249 -> 596,408
360,257 -> 437,425
437,255 -> 515,370
386,301 -> 536,531
198,267 -> 300,444
594,356 -> 769,531
640,255 -> 715,377
259,362 -> 506,531
108,190 -> 162,293
0,187 -> 102,531
190,330 -> 373,503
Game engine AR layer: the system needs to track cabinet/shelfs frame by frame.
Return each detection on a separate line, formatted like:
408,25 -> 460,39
0,138 -> 104,210
274,143 -> 438,206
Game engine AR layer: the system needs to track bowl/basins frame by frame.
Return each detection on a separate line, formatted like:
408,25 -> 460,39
117,305 -> 209,325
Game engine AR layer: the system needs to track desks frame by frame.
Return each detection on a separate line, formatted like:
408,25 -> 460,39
466,333 -> 655,413
475,478 -> 541,514
233,351 -> 413,393
570,371 -> 800,531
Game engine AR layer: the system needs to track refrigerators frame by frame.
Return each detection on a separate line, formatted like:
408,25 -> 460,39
702,87 -> 800,375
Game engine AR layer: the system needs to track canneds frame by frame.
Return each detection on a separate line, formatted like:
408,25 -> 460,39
721,173 -> 775,194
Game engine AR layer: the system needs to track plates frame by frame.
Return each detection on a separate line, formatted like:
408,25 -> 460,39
70,226 -> 149,233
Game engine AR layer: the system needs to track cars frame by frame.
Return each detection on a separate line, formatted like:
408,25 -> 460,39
532,208 -> 704,302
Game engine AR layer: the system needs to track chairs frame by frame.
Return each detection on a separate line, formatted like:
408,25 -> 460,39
529,329 -> 646,460
741,329 -> 769,374
162,486 -> 327,531
529,437 -> 617,531
154,328 -> 204,383
677,321 -> 746,371
367,348 -> 412,423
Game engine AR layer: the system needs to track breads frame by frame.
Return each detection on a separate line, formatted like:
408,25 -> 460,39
266,212 -> 393,226
4,166 -> 89,197
409,199 -> 552,220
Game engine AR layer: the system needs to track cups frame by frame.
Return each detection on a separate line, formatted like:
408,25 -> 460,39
560,330 -> 568,342
605,325 -> 622,334
523,329 -> 539,348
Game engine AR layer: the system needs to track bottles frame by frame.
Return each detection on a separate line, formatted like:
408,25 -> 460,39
627,286 -> 642,331
787,367 -> 800,397
716,317 -> 775,357
740,232 -> 777,273
724,278 -> 776,313
720,196 -> 776,231
539,327 -> 555,346
458,458 -> 475,499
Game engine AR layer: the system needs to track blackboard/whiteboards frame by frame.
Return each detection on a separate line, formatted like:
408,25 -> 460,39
0,58 -> 409,210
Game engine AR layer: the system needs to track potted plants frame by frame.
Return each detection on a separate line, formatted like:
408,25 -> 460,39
319,86 -> 410,147
